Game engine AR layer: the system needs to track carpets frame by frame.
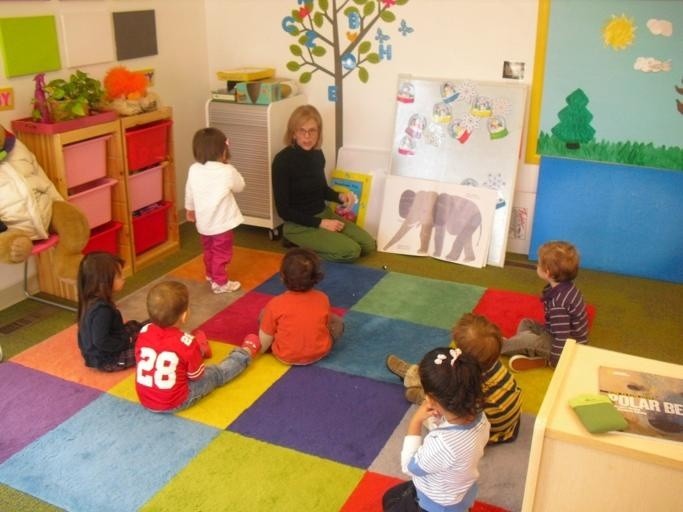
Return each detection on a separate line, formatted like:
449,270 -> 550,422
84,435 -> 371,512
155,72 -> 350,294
1,243 -> 598,510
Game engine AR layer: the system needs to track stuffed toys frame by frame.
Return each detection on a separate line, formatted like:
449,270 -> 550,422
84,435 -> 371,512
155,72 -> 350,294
0,124 -> 90,265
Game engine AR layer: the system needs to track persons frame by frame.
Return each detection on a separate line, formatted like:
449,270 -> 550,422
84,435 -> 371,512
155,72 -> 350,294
76,250 -> 152,373
382,346 -> 494,512
384,312 -> 526,447
271,103 -> 376,264
257,246 -> 346,368
499,239 -> 590,373
134,279 -> 263,413
182,127 -> 246,293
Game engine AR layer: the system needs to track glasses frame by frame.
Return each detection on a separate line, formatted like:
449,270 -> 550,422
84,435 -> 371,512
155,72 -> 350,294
297,129 -> 317,136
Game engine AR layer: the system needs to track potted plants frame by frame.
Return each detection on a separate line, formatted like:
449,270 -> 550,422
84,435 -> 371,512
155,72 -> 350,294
26,69 -> 105,123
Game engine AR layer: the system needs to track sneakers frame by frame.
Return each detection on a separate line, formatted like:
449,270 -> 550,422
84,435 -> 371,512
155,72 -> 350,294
206,276 -> 241,294
509,355 -> 546,373
191,329 -> 211,358
242,333 -> 260,358
387,355 -> 411,381
405,387 -> 426,405
328,322 -> 345,340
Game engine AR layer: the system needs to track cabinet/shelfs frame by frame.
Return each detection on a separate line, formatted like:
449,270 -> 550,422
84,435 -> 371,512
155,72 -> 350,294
10,106 -> 181,303
